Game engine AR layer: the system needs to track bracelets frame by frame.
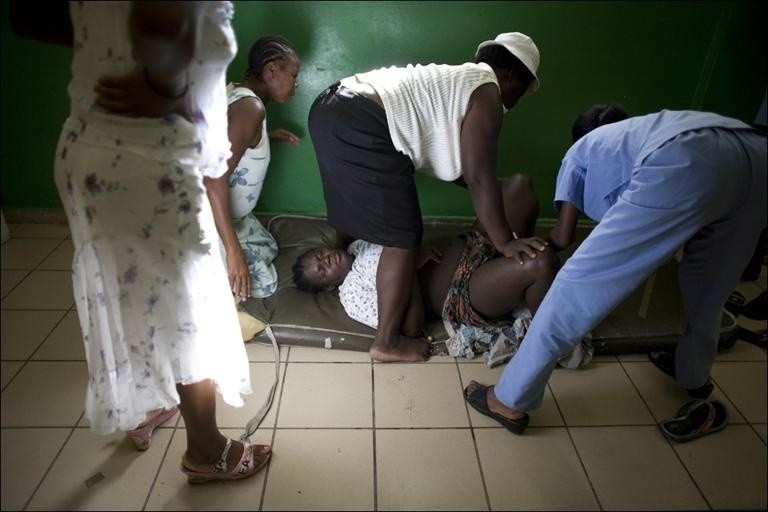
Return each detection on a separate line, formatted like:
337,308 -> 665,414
141,67 -> 188,101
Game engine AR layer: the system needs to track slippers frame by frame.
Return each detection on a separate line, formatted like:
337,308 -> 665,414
462,378 -> 529,435
649,344 -> 715,399
661,399 -> 732,442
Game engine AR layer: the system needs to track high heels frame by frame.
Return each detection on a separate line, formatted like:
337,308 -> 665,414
124,401 -> 180,450
177,437 -> 272,484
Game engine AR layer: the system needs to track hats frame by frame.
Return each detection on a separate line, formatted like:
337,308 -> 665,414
473,29 -> 540,96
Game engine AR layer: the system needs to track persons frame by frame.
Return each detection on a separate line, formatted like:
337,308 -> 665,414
462,102 -> 768,435
308,31 -> 552,363
24,1 -> 273,483
292,169 -> 560,328
202,33 -> 301,302
